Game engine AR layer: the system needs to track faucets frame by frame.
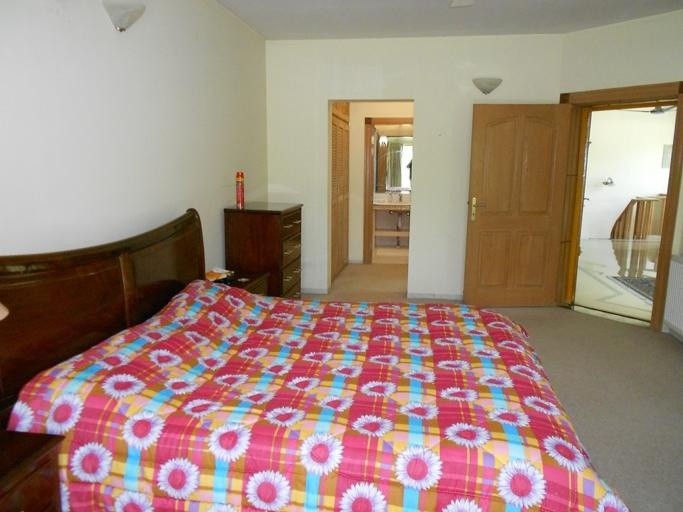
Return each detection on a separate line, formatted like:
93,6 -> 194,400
399,193 -> 403,201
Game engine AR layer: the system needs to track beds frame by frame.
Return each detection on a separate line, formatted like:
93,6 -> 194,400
0,208 -> 627,512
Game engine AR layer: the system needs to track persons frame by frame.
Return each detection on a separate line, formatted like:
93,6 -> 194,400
405,159 -> 411,187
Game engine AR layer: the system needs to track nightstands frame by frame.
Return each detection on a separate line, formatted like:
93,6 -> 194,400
0,429 -> 67,512
214,270 -> 273,296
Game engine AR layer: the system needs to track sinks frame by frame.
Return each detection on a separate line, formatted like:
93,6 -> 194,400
374,199 -> 410,212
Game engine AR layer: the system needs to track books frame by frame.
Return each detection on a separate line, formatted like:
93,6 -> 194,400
205,267 -> 234,281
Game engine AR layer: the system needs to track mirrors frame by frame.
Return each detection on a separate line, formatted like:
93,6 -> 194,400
373,135 -> 412,192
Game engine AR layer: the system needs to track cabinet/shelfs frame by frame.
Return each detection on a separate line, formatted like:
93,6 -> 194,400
330,115 -> 348,282
223,201 -> 303,298
331,100 -> 348,120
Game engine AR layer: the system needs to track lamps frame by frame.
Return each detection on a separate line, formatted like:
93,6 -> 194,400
472,77 -> 502,95
102,1 -> 147,34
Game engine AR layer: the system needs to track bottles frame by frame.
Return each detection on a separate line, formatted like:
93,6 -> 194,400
235,171 -> 244,207
